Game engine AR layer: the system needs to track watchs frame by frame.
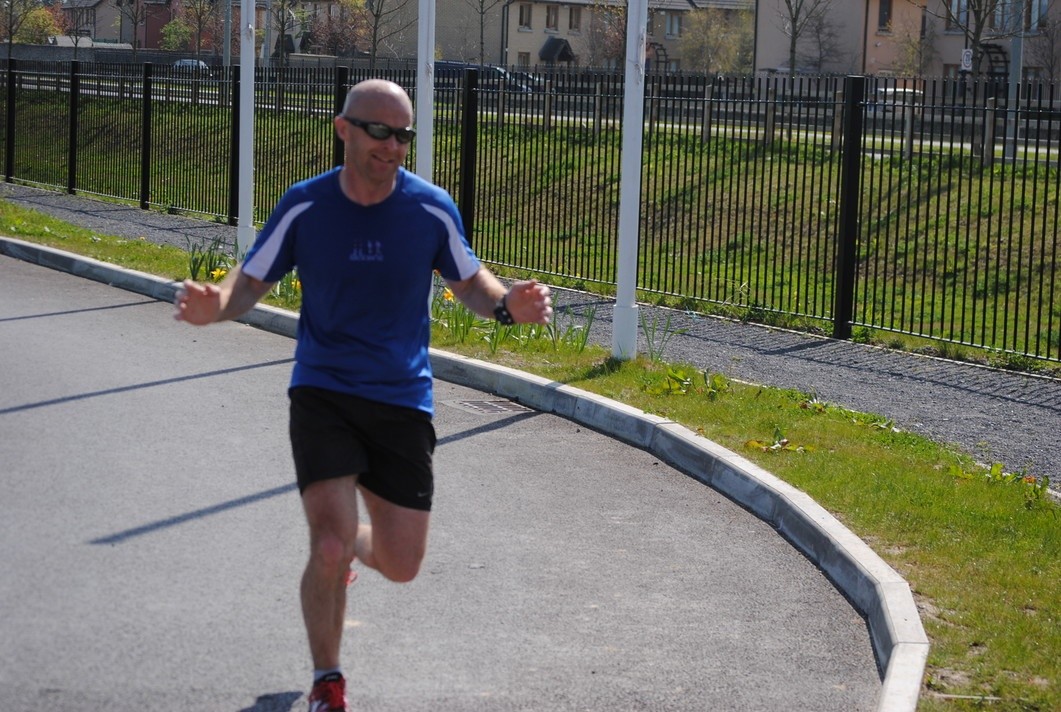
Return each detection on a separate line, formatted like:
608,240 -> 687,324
493,293 -> 516,325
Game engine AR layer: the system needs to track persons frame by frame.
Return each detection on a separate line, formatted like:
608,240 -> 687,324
172,78 -> 554,712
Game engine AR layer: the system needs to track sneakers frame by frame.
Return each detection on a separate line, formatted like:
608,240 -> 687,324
308,673 -> 346,712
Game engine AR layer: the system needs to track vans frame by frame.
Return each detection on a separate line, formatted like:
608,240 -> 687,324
871,87 -> 923,114
430,61 -> 530,102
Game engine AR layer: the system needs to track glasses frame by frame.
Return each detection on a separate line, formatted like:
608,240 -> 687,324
338,112 -> 417,143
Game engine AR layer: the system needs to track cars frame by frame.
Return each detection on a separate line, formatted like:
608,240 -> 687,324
505,71 -> 547,91
168,59 -> 212,79
